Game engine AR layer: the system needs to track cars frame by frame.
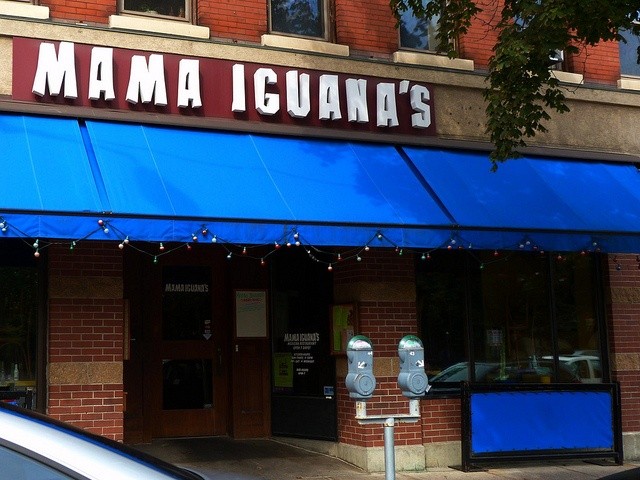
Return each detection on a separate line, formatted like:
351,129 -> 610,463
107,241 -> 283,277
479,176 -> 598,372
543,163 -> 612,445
542,356 -> 602,383
428,360 -> 580,384
0,403 -> 251,480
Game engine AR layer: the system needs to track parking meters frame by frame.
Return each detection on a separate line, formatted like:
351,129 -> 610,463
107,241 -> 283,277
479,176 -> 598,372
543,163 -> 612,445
346,334 -> 432,479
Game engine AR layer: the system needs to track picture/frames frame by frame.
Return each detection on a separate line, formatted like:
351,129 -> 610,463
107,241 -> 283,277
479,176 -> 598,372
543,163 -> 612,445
233,289 -> 270,340
328,300 -> 361,355
